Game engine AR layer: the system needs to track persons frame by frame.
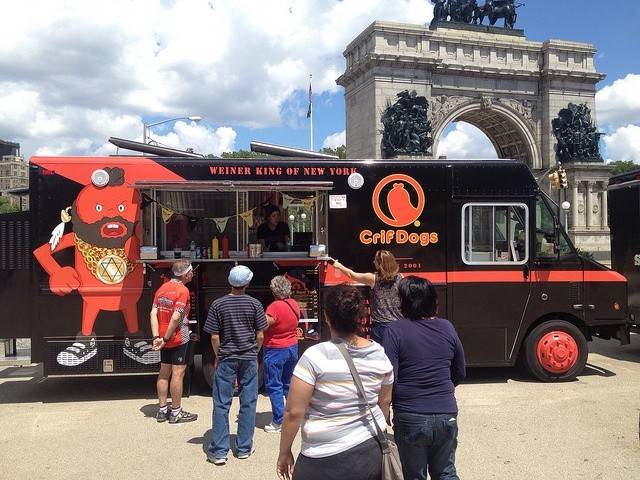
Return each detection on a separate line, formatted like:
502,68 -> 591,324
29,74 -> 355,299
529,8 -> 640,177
276,282 -> 394,480
256,205 -> 290,252
330,249 -> 405,346
382,275 -> 468,480
263,275 -> 301,434
149,259 -> 198,424
203,265 -> 270,465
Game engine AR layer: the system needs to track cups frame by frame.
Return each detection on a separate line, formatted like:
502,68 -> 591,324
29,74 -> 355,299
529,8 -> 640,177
255,243 -> 262,258
248,243 -> 256,258
172,247 -> 183,259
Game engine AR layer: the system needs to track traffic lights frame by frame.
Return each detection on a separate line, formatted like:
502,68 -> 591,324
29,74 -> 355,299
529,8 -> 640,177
548,172 -> 559,189
559,171 -> 568,189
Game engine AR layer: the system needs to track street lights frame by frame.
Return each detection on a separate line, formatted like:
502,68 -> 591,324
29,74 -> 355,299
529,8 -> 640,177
562,202 -> 570,252
143,116 -> 201,155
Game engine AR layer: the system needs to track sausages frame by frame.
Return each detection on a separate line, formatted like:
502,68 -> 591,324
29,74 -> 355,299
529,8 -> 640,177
386,183 -> 418,221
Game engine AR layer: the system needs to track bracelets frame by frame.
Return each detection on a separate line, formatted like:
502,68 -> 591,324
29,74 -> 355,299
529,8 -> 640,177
163,337 -> 168,342
152,336 -> 160,341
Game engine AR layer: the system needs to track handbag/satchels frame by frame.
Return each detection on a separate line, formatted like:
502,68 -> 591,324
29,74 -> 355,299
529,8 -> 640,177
381,433 -> 404,479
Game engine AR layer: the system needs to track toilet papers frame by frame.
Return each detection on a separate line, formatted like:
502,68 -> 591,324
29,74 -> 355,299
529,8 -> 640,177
140,246 -> 158,259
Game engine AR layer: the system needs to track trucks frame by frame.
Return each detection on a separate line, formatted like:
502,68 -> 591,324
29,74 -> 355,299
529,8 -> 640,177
0,156 -> 628,382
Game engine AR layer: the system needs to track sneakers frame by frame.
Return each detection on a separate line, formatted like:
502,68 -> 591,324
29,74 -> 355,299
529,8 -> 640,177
57,331 -> 97,366
208,454 -> 226,464
156,409 -> 168,422
123,331 -> 162,364
168,409 -> 198,423
264,421 -> 281,432
237,445 -> 255,458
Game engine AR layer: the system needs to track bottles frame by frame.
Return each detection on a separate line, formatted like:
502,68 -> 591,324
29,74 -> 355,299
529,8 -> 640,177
195,246 -> 201,259
211,235 -> 219,259
190,240 -> 197,259
221,235 -> 229,259
201,246 -> 207,259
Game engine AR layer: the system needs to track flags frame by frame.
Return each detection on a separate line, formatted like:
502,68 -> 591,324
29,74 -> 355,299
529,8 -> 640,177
306,82 -> 312,117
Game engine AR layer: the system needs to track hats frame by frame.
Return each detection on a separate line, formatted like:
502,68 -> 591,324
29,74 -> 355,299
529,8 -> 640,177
229,265 -> 255,288
264,204 -> 280,220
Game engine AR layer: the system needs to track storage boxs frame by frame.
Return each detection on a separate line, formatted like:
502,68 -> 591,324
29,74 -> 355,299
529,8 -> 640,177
140,246 -> 159,260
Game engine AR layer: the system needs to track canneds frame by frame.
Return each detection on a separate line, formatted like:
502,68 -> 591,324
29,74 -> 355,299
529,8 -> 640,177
202,247 -> 207,259
196,247 -> 201,258
